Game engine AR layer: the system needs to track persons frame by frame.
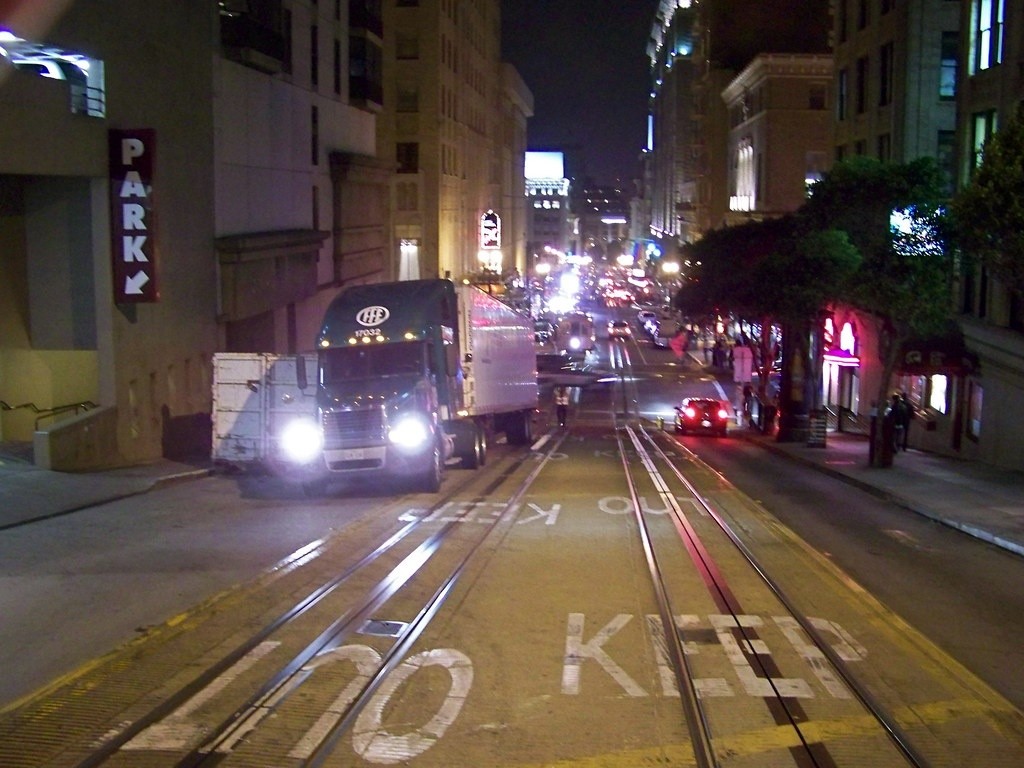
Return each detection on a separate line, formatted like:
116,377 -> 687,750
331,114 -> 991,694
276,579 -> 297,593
868,393 -> 914,455
742,382 -> 753,410
554,385 -> 569,426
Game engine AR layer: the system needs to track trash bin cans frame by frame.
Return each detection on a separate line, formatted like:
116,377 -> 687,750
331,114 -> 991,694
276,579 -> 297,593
869,415 -> 895,468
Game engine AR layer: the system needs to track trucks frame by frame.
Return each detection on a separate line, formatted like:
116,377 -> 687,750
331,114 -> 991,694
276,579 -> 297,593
555,311 -> 595,355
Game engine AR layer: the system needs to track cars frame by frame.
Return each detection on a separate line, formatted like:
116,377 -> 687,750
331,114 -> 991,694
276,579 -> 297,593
637,309 -> 681,352
566,260 -> 654,307
606,319 -> 632,340
674,396 -> 729,436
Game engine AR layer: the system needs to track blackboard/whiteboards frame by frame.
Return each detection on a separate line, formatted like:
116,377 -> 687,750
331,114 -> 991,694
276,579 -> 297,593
807,410 -> 827,447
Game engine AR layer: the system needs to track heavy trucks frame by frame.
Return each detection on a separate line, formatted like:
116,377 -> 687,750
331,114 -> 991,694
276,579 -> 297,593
294,276 -> 541,495
